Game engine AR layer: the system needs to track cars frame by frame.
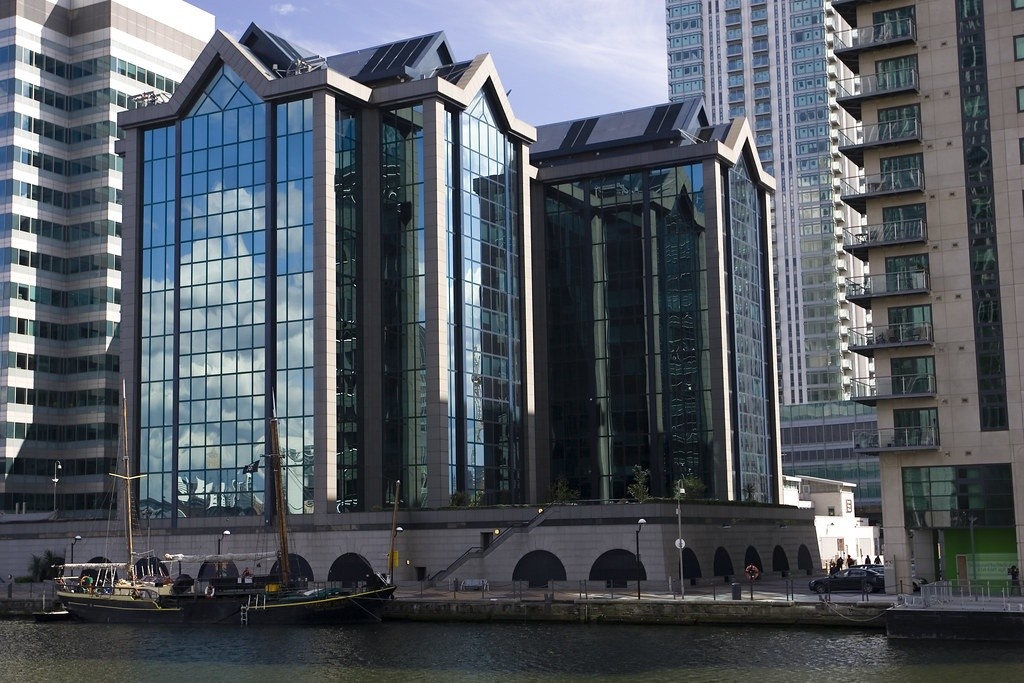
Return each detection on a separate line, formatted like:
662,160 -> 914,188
809,568 -> 884,594
912,577 -> 929,593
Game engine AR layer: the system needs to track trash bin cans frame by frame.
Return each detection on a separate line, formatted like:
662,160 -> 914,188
732,583 -> 741,599
366,574 -> 387,590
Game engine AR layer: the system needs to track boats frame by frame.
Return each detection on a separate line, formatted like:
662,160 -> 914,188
32,608 -> 76,621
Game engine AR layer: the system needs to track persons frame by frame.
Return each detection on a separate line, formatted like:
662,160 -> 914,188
865,554 -> 871,563
845,555 -> 854,568
873,553 -> 882,562
1011,565 -> 1021,596
836,556 -> 844,569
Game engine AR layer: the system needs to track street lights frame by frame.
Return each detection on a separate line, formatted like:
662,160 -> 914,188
389,527 -> 404,586
71,535 -> 82,577
52,460 -> 63,510
635,518 -> 646,600
218,529 -> 231,555
677,477 -> 686,599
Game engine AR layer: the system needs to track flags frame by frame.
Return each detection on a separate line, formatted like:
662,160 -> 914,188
242,459 -> 260,474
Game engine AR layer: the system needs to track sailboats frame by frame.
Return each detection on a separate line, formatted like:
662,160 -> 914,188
55,377 -> 397,624
